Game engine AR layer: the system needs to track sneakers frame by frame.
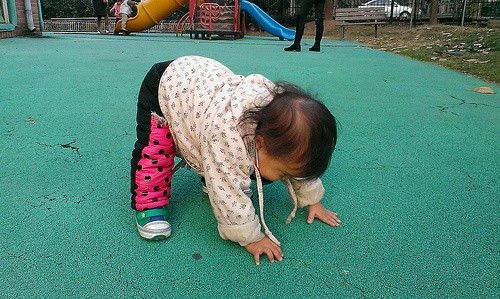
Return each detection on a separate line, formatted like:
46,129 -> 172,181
135,208 -> 172,241
201,177 -> 253,199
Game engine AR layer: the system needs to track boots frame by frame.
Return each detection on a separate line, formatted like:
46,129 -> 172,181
284,17 -> 306,52
309,18 -> 324,51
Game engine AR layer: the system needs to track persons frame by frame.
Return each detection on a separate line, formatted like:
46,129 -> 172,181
93,0 -> 110,33
129,55 -> 341,267
284,0 -> 326,52
110,0 -> 124,29
119,0 -> 139,31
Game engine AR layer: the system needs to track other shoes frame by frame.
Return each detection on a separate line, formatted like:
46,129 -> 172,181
98,30 -> 103,34
122,28 -> 128,32
106,29 -> 110,33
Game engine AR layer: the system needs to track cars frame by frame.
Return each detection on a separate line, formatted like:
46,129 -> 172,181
358,0 -> 422,21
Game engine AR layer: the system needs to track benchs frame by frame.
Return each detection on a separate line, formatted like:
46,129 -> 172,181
335,6 -> 388,39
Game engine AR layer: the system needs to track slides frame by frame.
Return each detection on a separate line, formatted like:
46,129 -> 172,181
241,1 -> 296,40
114,0 -> 188,33
